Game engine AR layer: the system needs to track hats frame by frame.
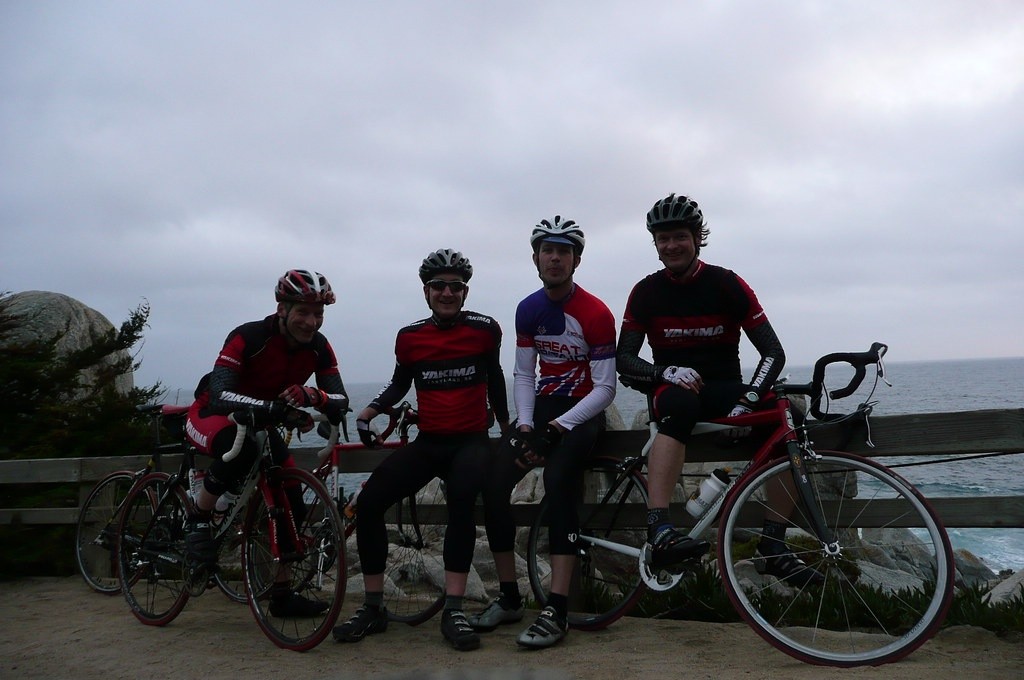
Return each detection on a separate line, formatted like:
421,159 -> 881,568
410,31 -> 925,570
541,235 -> 576,246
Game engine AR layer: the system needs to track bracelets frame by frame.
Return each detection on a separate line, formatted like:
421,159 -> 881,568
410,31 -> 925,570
317,390 -> 328,408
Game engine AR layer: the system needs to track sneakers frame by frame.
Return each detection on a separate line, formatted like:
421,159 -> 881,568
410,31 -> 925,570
517,606 -> 570,647
647,526 -> 694,556
441,609 -> 480,650
333,603 -> 388,641
181,513 -> 218,562
269,593 -> 329,618
466,595 -> 526,633
753,543 -> 828,587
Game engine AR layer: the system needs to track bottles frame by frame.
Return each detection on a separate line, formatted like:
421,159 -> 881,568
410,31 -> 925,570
212,482 -> 241,525
343,481 -> 366,519
194,469 -> 205,499
682,466 -> 731,519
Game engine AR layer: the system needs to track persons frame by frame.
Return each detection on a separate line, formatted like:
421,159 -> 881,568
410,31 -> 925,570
464,214 -> 617,651
617,192 -> 823,589
331,248 -> 514,654
183,268 -> 348,618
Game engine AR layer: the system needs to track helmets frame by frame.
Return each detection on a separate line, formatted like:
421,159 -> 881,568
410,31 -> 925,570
419,249 -> 472,286
647,193 -> 702,233
275,270 -> 335,304
531,216 -> 584,256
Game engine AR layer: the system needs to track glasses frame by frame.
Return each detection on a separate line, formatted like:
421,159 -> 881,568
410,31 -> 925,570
426,279 -> 466,293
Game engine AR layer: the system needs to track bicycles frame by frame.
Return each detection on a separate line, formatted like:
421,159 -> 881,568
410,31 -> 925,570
72,398 -> 447,653
526,341 -> 956,668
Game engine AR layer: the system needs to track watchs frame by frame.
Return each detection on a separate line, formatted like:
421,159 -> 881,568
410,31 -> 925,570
743,391 -> 760,405
